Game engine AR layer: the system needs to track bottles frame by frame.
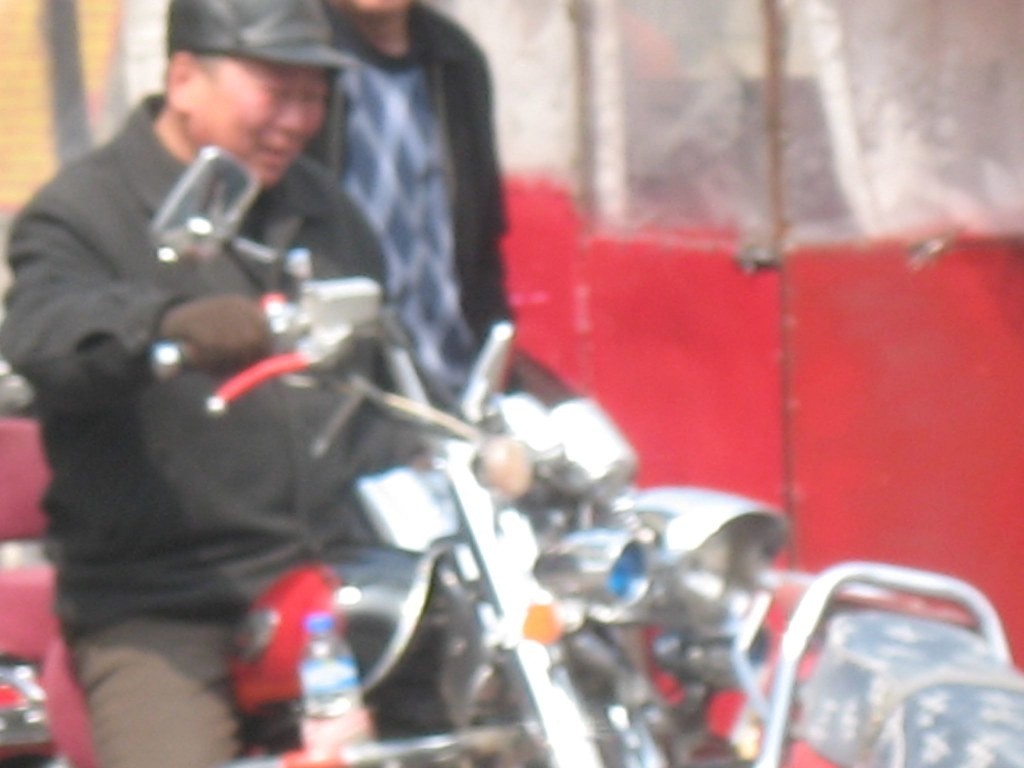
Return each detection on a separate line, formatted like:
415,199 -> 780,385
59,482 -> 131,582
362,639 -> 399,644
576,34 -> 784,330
294,613 -> 360,720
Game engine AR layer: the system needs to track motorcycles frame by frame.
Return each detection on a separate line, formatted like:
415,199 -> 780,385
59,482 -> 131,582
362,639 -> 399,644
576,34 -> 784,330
0,148 -> 1024,767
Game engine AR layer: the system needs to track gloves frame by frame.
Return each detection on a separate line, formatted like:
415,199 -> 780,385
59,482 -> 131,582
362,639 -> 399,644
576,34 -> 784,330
159,298 -> 274,375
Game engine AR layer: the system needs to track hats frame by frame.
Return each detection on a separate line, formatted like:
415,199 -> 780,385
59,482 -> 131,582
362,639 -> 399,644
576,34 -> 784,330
168,0 -> 360,67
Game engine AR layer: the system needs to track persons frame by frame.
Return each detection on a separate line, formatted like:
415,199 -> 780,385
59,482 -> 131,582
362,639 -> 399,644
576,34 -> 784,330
1,0 -> 531,768
299,0 -> 519,420
